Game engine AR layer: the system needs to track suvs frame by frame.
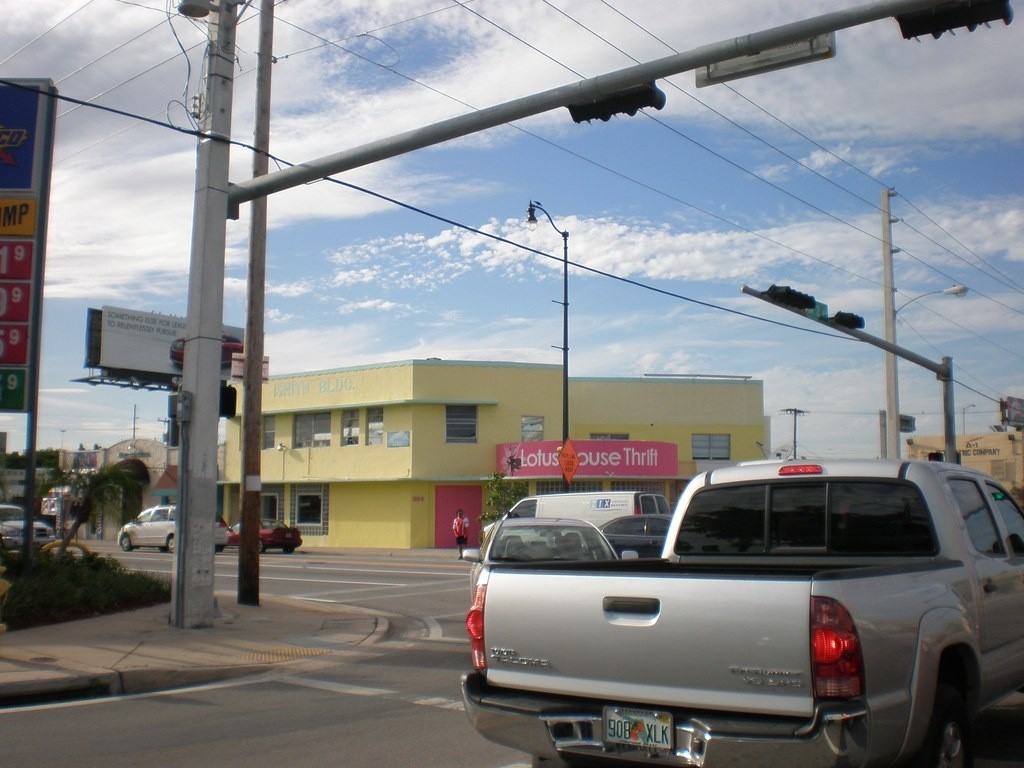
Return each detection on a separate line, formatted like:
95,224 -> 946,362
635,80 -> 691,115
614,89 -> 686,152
116,505 -> 229,554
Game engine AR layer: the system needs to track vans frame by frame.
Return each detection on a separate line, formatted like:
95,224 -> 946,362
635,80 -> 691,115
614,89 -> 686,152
482,491 -> 672,549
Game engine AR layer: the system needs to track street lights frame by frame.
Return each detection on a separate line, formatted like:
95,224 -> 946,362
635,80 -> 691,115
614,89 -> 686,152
962,403 -> 976,436
886,285 -> 969,458
525,198 -> 579,493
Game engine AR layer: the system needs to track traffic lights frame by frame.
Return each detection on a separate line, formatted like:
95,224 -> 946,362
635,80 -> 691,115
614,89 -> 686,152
767,284 -> 816,309
992,492 -> 1005,500
835,311 -> 865,330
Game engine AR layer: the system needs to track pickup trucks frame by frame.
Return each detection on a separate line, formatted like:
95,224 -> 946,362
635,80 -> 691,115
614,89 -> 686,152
460,458 -> 1024,768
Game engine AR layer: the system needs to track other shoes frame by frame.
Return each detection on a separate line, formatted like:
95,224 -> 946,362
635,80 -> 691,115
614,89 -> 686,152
458,556 -> 463,560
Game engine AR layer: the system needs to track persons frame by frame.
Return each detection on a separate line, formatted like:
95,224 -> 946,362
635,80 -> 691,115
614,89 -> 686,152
453,509 -> 470,560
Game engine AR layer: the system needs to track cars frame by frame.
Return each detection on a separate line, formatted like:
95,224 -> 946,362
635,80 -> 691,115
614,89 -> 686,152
226,518 -> 303,554
170,334 -> 243,368
461,514 -> 670,601
0,505 -> 55,549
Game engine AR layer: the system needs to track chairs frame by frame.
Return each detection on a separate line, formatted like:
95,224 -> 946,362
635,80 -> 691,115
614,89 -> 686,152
504,537 -> 523,558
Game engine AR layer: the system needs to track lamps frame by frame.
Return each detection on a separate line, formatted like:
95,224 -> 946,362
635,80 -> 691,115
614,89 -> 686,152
277,442 -> 287,451
